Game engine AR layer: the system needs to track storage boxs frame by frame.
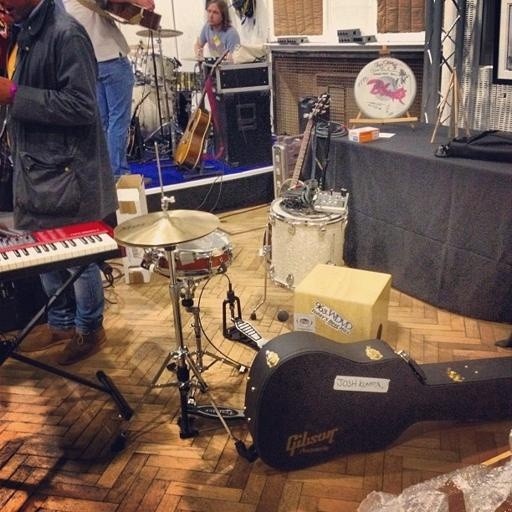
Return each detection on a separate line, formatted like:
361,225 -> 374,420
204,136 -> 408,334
294,262 -> 392,343
114,173 -> 149,226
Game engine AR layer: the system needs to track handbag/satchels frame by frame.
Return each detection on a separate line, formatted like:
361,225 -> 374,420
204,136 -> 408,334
435,129 -> 512,164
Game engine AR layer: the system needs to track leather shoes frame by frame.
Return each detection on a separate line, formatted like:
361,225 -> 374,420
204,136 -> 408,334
19,325 -> 76,352
56,327 -> 108,365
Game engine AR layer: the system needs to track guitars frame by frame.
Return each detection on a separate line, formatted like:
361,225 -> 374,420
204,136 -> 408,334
105,1 -> 163,29
175,49 -> 229,173
263,92 -> 331,266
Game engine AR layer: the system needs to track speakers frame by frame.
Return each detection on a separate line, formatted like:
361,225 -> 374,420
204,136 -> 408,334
212,85 -> 272,166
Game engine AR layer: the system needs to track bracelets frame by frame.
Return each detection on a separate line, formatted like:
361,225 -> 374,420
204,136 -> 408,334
9,82 -> 17,103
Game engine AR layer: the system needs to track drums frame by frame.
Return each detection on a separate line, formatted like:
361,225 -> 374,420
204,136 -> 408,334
168,71 -> 199,93
269,193 -> 349,290
130,52 -> 182,87
140,229 -> 233,278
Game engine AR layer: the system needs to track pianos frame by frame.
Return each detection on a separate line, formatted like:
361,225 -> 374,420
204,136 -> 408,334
0,220 -> 120,283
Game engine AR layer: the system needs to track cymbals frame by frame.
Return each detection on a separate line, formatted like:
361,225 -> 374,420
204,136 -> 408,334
113,210 -> 221,248
135,29 -> 182,36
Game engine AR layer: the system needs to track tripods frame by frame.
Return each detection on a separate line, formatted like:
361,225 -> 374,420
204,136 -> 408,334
167,308 -> 247,399
138,32 -> 184,167
110,247 -> 247,455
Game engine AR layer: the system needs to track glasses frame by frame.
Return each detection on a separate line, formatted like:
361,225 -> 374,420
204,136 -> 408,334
1,7 -> 16,17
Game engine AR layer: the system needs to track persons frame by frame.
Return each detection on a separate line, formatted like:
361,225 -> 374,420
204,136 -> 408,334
0,1 -> 120,367
190,0 -> 242,64
64,2 -> 138,179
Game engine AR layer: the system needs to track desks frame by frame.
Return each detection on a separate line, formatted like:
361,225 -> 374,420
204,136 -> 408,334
326,121 -> 511,323
